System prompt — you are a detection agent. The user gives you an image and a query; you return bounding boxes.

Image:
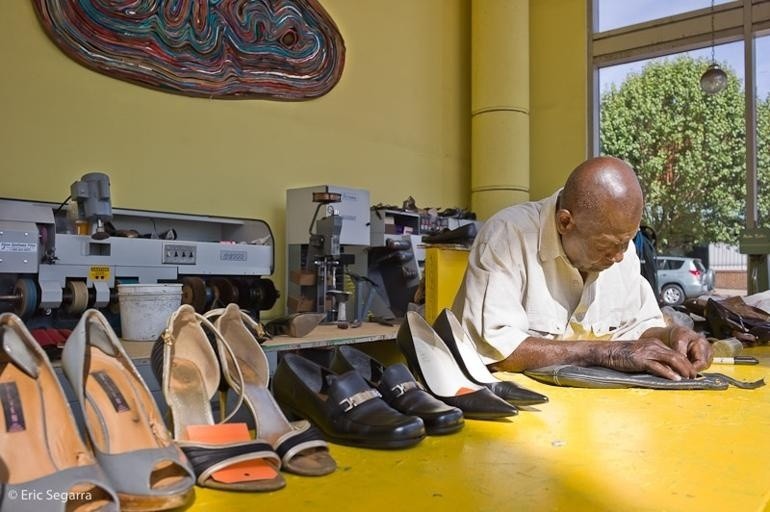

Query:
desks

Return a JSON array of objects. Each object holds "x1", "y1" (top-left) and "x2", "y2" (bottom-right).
[{"x1": 4, "y1": 320, "x2": 404, "y2": 458}]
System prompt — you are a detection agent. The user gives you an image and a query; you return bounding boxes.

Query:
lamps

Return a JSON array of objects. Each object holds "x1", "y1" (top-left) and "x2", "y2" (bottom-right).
[{"x1": 700, "y1": 1, "x2": 727, "y2": 94}]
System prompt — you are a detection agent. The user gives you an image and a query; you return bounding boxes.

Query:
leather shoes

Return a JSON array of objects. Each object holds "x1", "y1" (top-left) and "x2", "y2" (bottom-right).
[
  {"x1": 403, "y1": 195, "x2": 477, "y2": 219},
  {"x1": 272, "y1": 352, "x2": 427, "y2": 450},
  {"x1": 329, "y1": 345, "x2": 465, "y2": 435},
  {"x1": 420, "y1": 223, "x2": 476, "y2": 249}
]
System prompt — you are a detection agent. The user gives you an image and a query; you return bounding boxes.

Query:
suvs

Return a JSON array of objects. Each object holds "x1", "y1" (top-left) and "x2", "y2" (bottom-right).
[{"x1": 654, "y1": 256, "x2": 715, "y2": 306}]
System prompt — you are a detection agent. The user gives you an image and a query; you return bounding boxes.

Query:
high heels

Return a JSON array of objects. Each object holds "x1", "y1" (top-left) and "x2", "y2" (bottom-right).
[
  {"x1": 151, "y1": 304, "x2": 287, "y2": 492},
  {"x1": 0, "y1": 310, "x2": 121, "y2": 512},
  {"x1": 61, "y1": 309, "x2": 196, "y2": 511},
  {"x1": 705, "y1": 298, "x2": 770, "y2": 342},
  {"x1": 396, "y1": 310, "x2": 519, "y2": 418},
  {"x1": 211, "y1": 303, "x2": 337, "y2": 476},
  {"x1": 264, "y1": 312, "x2": 328, "y2": 336},
  {"x1": 433, "y1": 309, "x2": 549, "y2": 405}
]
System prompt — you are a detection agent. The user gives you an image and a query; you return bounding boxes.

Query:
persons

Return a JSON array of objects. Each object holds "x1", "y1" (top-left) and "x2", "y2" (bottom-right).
[{"x1": 451, "y1": 156, "x2": 715, "y2": 381}]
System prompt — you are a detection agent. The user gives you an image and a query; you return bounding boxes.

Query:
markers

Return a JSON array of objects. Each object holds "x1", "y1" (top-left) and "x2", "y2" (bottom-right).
[{"x1": 710, "y1": 355, "x2": 760, "y2": 366}]
